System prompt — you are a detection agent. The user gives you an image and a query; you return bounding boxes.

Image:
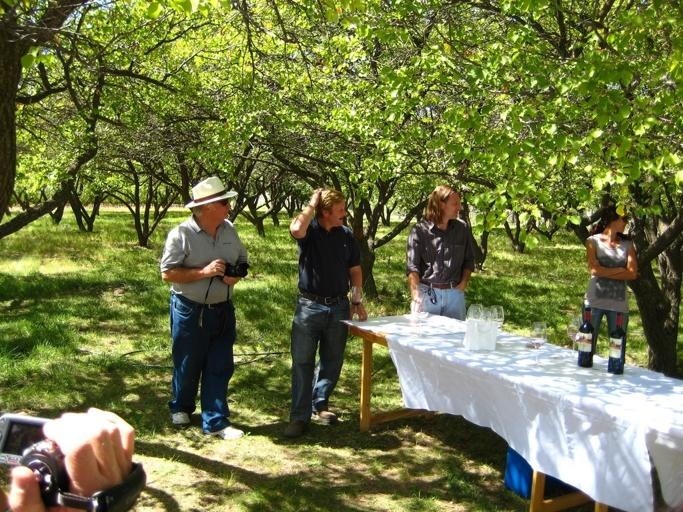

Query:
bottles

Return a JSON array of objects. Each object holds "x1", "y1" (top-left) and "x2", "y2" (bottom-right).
[
  {"x1": 607, "y1": 311, "x2": 626, "y2": 375},
  {"x1": 577, "y1": 307, "x2": 595, "y2": 368}
]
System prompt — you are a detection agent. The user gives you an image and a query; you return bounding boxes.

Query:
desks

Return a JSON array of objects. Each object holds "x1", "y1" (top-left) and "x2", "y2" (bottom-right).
[{"x1": 336, "y1": 309, "x2": 682, "y2": 511}]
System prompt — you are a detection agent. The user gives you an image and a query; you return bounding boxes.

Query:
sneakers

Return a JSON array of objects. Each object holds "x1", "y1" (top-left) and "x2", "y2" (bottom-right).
[
  {"x1": 172, "y1": 412, "x2": 191, "y2": 424},
  {"x1": 207, "y1": 426, "x2": 244, "y2": 439},
  {"x1": 283, "y1": 422, "x2": 305, "y2": 437},
  {"x1": 314, "y1": 411, "x2": 337, "y2": 420}
]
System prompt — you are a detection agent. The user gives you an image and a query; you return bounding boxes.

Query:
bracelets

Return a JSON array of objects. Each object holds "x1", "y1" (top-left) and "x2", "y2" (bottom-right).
[{"x1": 351, "y1": 301, "x2": 360, "y2": 306}]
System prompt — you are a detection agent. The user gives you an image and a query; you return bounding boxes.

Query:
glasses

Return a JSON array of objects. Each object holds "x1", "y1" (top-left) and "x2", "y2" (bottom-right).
[{"x1": 220, "y1": 199, "x2": 229, "y2": 206}]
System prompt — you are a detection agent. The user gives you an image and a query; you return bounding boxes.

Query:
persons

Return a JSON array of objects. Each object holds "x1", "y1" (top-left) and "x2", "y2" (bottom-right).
[
  {"x1": 281, "y1": 187, "x2": 368, "y2": 438},
  {"x1": 406, "y1": 184, "x2": 475, "y2": 320},
  {"x1": 159, "y1": 175, "x2": 247, "y2": 440},
  {"x1": 584, "y1": 204, "x2": 637, "y2": 360},
  {"x1": 0, "y1": 406, "x2": 136, "y2": 511}
]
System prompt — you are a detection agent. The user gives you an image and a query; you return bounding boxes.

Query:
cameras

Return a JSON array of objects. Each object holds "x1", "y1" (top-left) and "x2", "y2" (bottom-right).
[
  {"x1": 0, "y1": 412, "x2": 69, "y2": 511},
  {"x1": 217, "y1": 262, "x2": 248, "y2": 280}
]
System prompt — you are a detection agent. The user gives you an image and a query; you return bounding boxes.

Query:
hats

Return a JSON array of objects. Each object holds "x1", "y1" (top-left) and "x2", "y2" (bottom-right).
[{"x1": 185, "y1": 176, "x2": 238, "y2": 208}]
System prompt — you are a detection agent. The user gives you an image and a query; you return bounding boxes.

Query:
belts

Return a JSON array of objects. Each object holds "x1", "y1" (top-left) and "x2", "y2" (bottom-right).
[
  {"x1": 421, "y1": 281, "x2": 459, "y2": 288},
  {"x1": 178, "y1": 295, "x2": 228, "y2": 309},
  {"x1": 303, "y1": 291, "x2": 346, "y2": 305}
]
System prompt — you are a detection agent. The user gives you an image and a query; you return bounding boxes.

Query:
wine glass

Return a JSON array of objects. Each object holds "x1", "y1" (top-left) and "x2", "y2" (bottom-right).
[
  {"x1": 462, "y1": 304, "x2": 504, "y2": 355},
  {"x1": 528, "y1": 321, "x2": 547, "y2": 368},
  {"x1": 567, "y1": 320, "x2": 581, "y2": 363}
]
